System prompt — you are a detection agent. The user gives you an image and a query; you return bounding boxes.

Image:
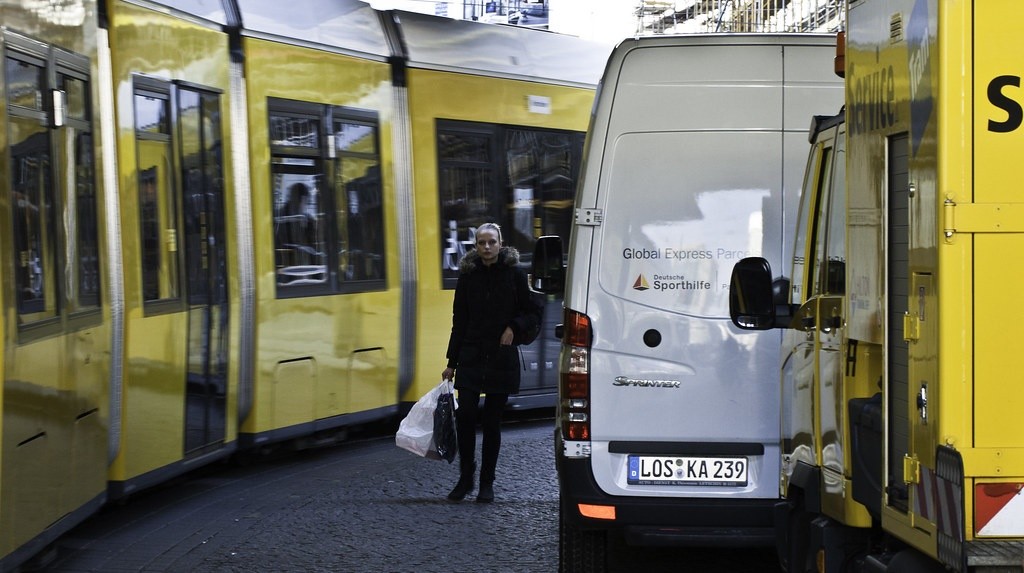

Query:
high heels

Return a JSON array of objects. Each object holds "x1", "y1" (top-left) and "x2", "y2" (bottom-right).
[
  {"x1": 478, "y1": 468, "x2": 496, "y2": 502},
  {"x1": 448, "y1": 464, "x2": 477, "y2": 500}
]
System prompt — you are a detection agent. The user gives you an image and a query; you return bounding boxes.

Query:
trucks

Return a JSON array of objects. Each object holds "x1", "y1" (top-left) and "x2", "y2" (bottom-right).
[{"x1": 729, "y1": 0, "x2": 1023, "y2": 573}]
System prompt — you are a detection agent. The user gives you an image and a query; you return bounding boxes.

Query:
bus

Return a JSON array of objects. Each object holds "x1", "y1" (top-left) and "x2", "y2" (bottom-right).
[{"x1": 0, "y1": 3, "x2": 613, "y2": 572}]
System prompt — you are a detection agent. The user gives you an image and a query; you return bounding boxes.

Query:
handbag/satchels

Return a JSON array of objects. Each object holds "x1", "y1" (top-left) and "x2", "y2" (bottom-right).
[{"x1": 395, "y1": 379, "x2": 458, "y2": 463}]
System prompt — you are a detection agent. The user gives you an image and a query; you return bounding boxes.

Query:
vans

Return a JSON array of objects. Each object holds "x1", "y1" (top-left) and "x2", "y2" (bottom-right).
[{"x1": 531, "y1": 33, "x2": 847, "y2": 573}]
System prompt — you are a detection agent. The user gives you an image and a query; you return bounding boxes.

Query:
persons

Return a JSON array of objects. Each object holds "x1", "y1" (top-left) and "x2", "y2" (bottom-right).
[{"x1": 440, "y1": 223, "x2": 544, "y2": 507}]
